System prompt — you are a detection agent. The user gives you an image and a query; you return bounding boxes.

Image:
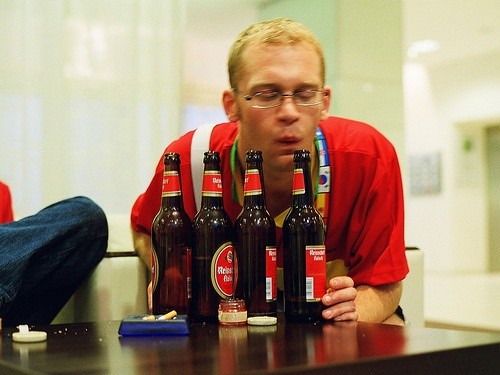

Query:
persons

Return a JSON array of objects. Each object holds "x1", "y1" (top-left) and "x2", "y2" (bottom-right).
[
  {"x1": 131, "y1": 18, "x2": 411, "y2": 321},
  {"x1": 0, "y1": 181, "x2": 109, "y2": 329}
]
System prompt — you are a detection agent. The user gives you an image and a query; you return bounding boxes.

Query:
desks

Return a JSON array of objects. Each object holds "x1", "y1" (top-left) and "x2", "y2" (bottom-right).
[{"x1": 0, "y1": 316, "x2": 500, "y2": 375}]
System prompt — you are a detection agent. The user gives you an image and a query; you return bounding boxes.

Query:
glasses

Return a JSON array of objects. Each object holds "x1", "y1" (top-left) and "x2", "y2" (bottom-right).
[{"x1": 236, "y1": 88, "x2": 327, "y2": 109}]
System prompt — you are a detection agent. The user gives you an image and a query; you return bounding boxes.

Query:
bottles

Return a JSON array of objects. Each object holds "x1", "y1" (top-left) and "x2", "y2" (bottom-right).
[
  {"x1": 281, "y1": 150, "x2": 327, "y2": 326},
  {"x1": 233, "y1": 149, "x2": 279, "y2": 319},
  {"x1": 192, "y1": 151, "x2": 238, "y2": 325},
  {"x1": 150, "y1": 153, "x2": 195, "y2": 323}
]
